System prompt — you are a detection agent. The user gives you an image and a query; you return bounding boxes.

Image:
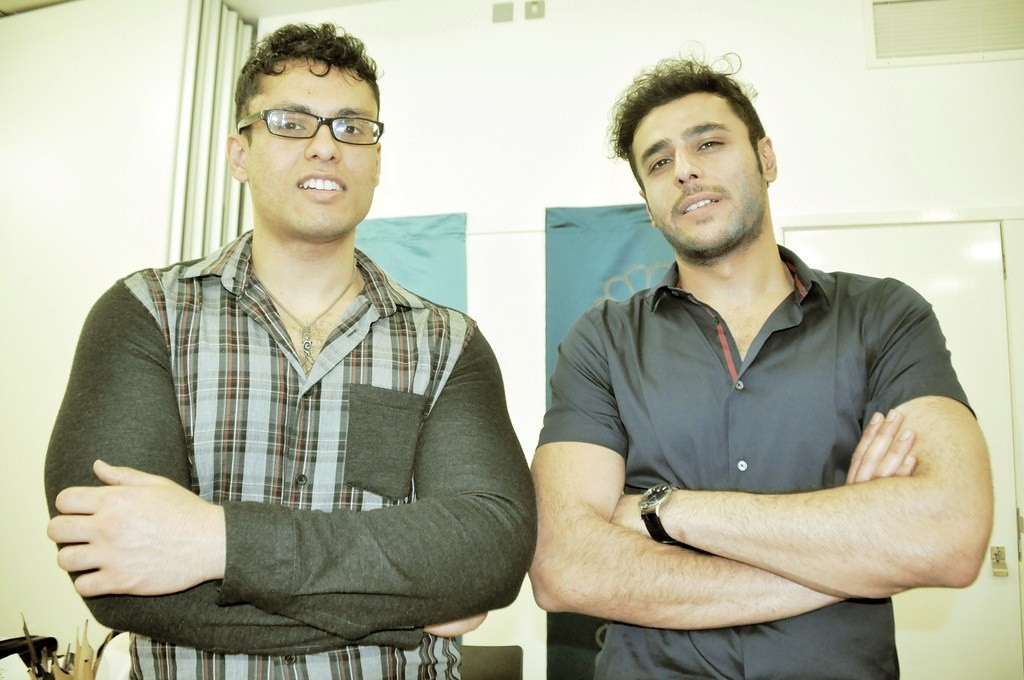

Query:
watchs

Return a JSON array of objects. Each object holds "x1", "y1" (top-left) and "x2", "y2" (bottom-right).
[{"x1": 638, "y1": 485, "x2": 679, "y2": 545}]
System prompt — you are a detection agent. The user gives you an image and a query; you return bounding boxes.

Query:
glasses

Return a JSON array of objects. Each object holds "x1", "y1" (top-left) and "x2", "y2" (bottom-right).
[{"x1": 238, "y1": 109, "x2": 384, "y2": 145}]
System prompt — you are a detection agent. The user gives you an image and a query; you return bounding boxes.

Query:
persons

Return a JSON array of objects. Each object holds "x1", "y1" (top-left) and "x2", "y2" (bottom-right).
[
  {"x1": 528, "y1": 62, "x2": 996, "y2": 680},
  {"x1": 44, "y1": 22, "x2": 537, "y2": 680}
]
